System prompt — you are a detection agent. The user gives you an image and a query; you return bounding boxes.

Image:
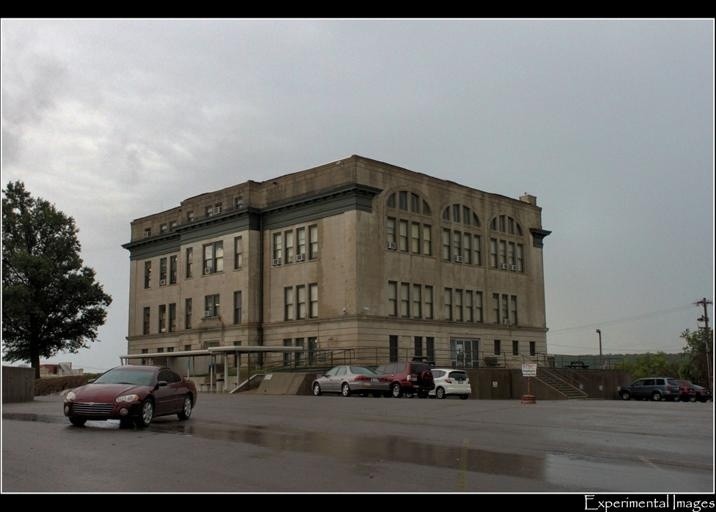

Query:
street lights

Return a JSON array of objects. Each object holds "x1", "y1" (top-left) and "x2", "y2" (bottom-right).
[{"x1": 596, "y1": 328, "x2": 602, "y2": 368}]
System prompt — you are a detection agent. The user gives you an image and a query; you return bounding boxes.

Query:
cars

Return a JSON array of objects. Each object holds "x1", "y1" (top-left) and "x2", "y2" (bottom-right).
[
  {"x1": 618, "y1": 377, "x2": 711, "y2": 402},
  {"x1": 564, "y1": 360, "x2": 589, "y2": 368},
  {"x1": 311, "y1": 362, "x2": 472, "y2": 399},
  {"x1": 64, "y1": 364, "x2": 198, "y2": 428}
]
